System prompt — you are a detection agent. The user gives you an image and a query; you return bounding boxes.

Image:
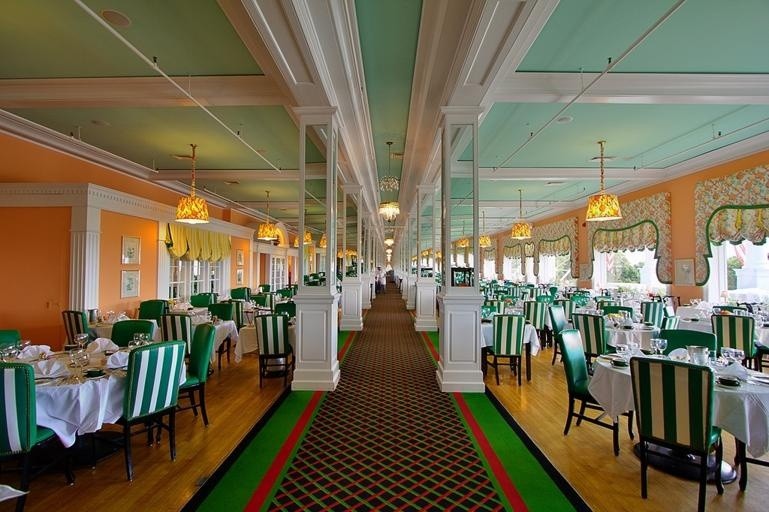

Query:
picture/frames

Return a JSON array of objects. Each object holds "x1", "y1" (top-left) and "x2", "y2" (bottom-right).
[
  {"x1": 121, "y1": 236, "x2": 141, "y2": 265},
  {"x1": 236, "y1": 249, "x2": 244, "y2": 266}
]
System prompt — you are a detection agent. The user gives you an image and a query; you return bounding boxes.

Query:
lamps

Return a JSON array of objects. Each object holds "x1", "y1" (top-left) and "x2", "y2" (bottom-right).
[
  {"x1": 455, "y1": 211, "x2": 491, "y2": 250},
  {"x1": 257, "y1": 190, "x2": 278, "y2": 241},
  {"x1": 294, "y1": 210, "x2": 326, "y2": 248},
  {"x1": 510, "y1": 189, "x2": 533, "y2": 240},
  {"x1": 176, "y1": 145, "x2": 211, "y2": 223},
  {"x1": 378, "y1": 142, "x2": 400, "y2": 224},
  {"x1": 585, "y1": 141, "x2": 623, "y2": 222}
]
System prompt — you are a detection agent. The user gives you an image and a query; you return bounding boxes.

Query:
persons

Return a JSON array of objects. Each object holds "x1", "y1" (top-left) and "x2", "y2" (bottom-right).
[{"x1": 376, "y1": 264, "x2": 388, "y2": 294}]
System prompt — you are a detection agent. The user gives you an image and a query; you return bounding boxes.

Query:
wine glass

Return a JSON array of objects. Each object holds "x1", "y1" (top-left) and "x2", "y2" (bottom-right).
[
  {"x1": 609, "y1": 338, "x2": 745, "y2": 390},
  {"x1": 0, "y1": 333, "x2": 151, "y2": 380}
]
